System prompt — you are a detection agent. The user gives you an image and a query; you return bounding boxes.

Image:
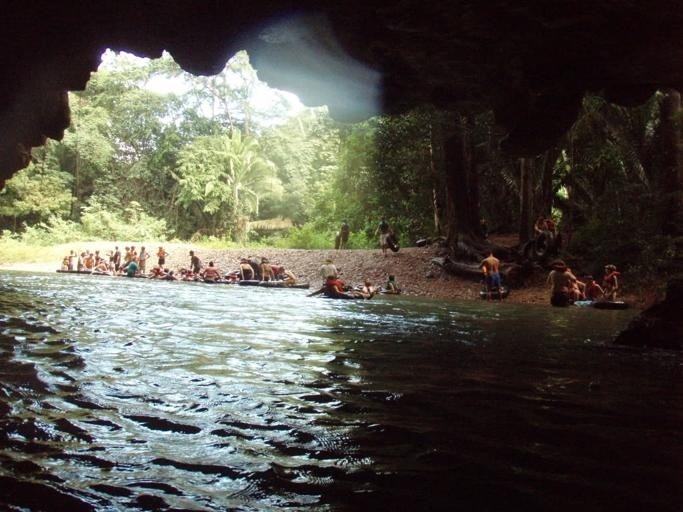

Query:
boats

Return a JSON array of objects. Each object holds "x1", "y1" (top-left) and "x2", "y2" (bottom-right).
[{"x1": 54, "y1": 267, "x2": 311, "y2": 288}]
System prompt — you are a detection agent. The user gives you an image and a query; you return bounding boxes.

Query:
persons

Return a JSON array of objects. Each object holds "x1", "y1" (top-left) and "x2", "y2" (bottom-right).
[
  {"x1": 374, "y1": 215, "x2": 393, "y2": 257},
  {"x1": 336, "y1": 276, "x2": 346, "y2": 292},
  {"x1": 478, "y1": 248, "x2": 503, "y2": 303},
  {"x1": 544, "y1": 258, "x2": 577, "y2": 306},
  {"x1": 566, "y1": 264, "x2": 620, "y2": 305},
  {"x1": 325, "y1": 275, "x2": 347, "y2": 299},
  {"x1": 501, "y1": 215, "x2": 557, "y2": 288},
  {"x1": 380, "y1": 274, "x2": 400, "y2": 294},
  {"x1": 339, "y1": 220, "x2": 349, "y2": 249},
  {"x1": 61, "y1": 246, "x2": 295, "y2": 287},
  {"x1": 360, "y1": 278, "x2": 374, "y2": 300},
  {"x1": 318, "y1": 257, "x2": 338, "y2": 284},
  {"x1": 334, "y1": 232, "x2": 341, "y2": 250}
]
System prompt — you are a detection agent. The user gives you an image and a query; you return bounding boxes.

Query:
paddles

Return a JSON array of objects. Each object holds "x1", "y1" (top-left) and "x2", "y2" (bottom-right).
[{"x1": 306, "y1": 287, "x2": 326, "y2": 296}]
